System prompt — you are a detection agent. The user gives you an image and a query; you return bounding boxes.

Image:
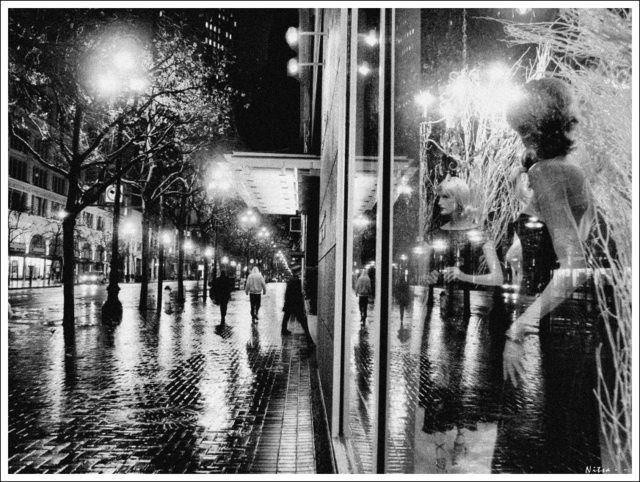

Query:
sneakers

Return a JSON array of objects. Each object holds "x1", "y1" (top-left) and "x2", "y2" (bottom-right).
[
  {"x1": 281, "y1": 329, "x2": 292, "y2": 335},
  {"x1": 252, "y1": 315, "x2": 259, "y2": 323}
]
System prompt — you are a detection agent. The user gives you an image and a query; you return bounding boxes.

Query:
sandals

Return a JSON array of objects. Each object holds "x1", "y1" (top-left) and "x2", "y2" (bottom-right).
[
  {"x1": 453, "y1": 430, "x2": 470, "y2": 466},
  {"x1": 434, "y1": 442, "x2": 448, "y2": 474}
]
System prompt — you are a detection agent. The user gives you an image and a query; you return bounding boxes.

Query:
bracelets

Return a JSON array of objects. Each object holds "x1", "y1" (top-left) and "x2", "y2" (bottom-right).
[{"x1": 503, "y1": 329, "x2": 526, "y2": 346}]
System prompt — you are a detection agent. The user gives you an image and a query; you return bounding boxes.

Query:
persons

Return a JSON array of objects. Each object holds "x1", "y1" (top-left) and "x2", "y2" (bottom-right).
[
  {"x1": 352, "y1": 265, "x2": 373, "y2": 329},
  {"x1": 415, "y1": 171, "x2": 505, "y2": 474},
  {"x1": 393, "y1": 274, "x2": 412, "y2": 326},
  {"x1": 211, "y1": 271, "x2": 235, "y2": 327},
  {"x1": 278, "y1": 264, "x2": 311, "y2": 337},
  {"x1": 244, "y1": 264, "x2": 267, "y2": 323},
  {"x1": 487, "y1": 74, "x2": 609, "y2": 475}
]
